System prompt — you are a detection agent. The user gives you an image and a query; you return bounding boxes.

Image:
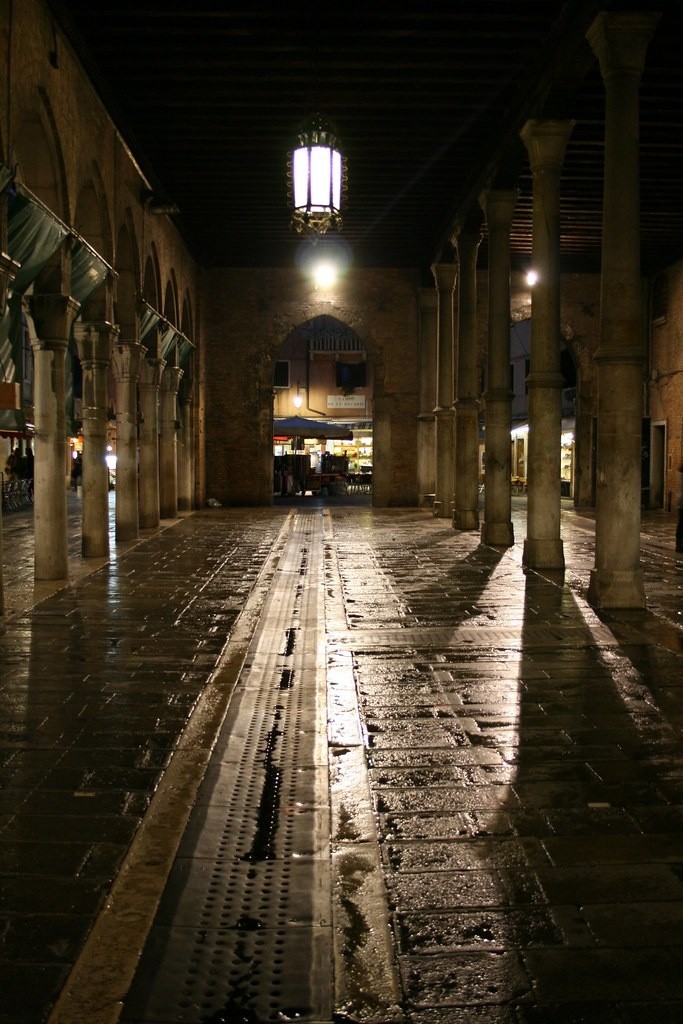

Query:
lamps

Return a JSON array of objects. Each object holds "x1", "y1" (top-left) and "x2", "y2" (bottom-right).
[{"x1": 286, "y1": 110, "x2": 349, "y2": 235}]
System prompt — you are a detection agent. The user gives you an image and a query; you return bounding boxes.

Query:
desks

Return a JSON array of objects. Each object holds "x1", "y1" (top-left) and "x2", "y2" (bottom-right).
[{"x1": 312, "y1": 473, "x2": 339, "y2": 496}]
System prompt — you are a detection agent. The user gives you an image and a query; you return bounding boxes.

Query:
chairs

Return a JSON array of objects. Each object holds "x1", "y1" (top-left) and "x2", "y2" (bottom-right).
[{"x1": 346, "y1": 474, "x2": 373, "y2": 495}]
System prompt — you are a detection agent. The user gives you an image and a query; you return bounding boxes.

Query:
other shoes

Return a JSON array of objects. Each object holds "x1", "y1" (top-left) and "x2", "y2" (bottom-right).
[{"x1": 72, "y1": 487, "x2": 76, "y2": 491}]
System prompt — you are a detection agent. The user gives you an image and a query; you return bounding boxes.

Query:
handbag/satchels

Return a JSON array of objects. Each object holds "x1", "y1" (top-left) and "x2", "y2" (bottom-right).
[{"x1": 8, "y1": 467, "x2": 13, "y2": 475}]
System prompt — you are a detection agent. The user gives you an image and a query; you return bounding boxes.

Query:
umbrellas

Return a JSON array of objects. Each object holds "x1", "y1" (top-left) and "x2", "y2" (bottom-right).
[{"x1": 274, "y1": 416, "x2": 353, "y2": 469}]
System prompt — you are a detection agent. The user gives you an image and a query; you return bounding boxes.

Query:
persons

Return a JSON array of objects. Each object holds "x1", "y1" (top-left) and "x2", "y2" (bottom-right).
[
  {"x1": 7, "y1": 448, "x2": 34, "y2": 496},
  {"x1": 72, "y1": 454, "x2": 81, "y2": 491},
  {"x1": 512, "y1": 476, "x2": 522, "y2": 495},
  {"x1": 342, "y1": 450, "x2": 349, "y2": 470}
]
